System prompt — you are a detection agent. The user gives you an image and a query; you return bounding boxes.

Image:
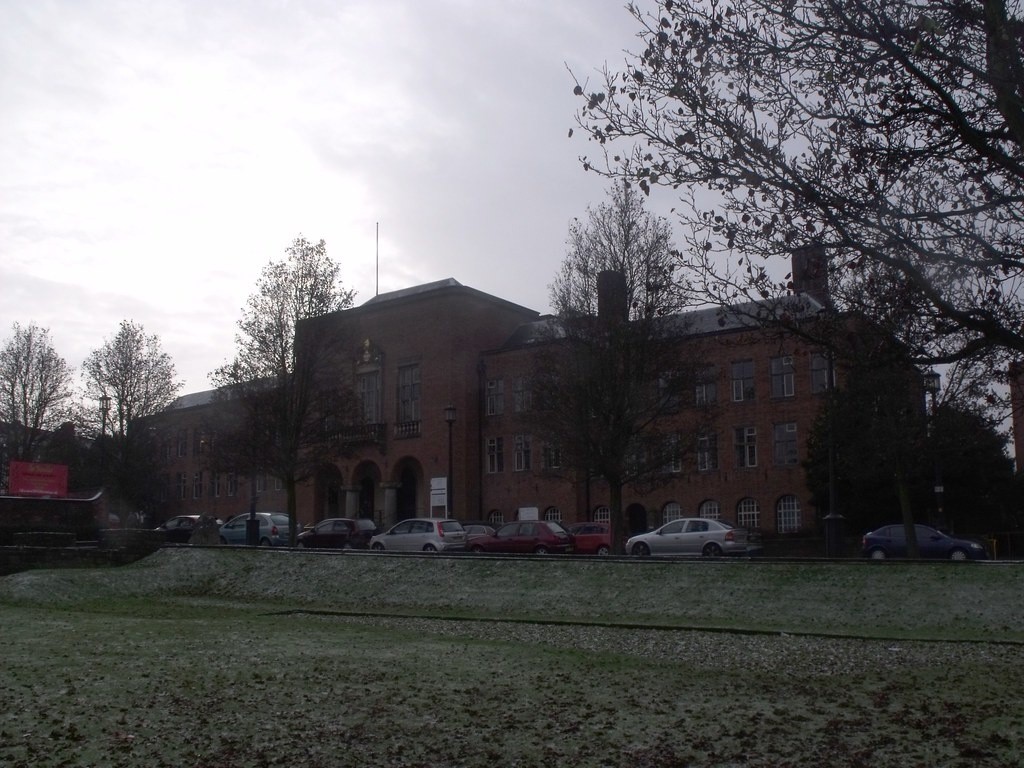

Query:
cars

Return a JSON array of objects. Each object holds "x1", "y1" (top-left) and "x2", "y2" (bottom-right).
[
  {"x1": 369, "y1": 516, "x2": 468, "y2": 552},
  {"x1": 465, "y1": 519, "x2": 577, "y2": 556},
  {"x1": 567, "y1": 522, "x2": 630, "y2": 557},
  {"x1": 219, "y1": 513, "x2": 301, "y2": 547},
  {"x1": 625, "y1": 517, "x2": 749, "y2": 560},
  {"x1": 462, "y1": 526, "x2": 498, "y2": 540},
  {"x1": 296, "y1": 517, "x2": 381, "y2": 549},
  {"x1": 862, "y1": 525, "x2": 987, "y2": 562},
  {"x1": 154, "y1": 514, "x2": 224, "y2": 542}
]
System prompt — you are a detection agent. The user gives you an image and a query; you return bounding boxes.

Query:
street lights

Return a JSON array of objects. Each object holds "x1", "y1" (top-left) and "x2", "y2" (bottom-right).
[
  {"x1": 925, "y1": 365, "x2": 942, "y2": 422},
  {"x1": 98, "y1": 396, "x2": 113, "y2": 485},
  {"x1": 818, "y1": 303, "x2": 849, "y2": 559},
  {"x1": 443, "y1": 404, "x2": 458, "y2": 518},
  {"x1": 245, "y1": 401, "x2": 264, "y2": 546}
]
